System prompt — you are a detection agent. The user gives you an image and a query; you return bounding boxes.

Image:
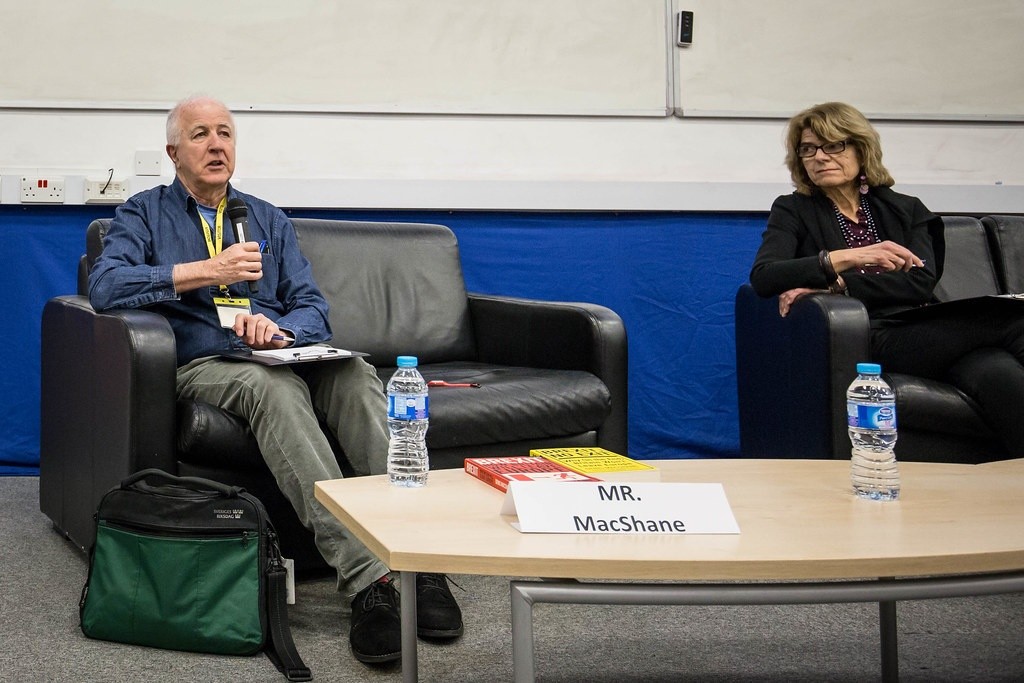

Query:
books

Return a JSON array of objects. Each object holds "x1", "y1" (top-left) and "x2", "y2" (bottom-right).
[
  {"x1": 464, "y1": 456, "x2": 606, "y2": 494},
  {"x1": 530, "y1": 447, "x2": 661, "y2": 482}
]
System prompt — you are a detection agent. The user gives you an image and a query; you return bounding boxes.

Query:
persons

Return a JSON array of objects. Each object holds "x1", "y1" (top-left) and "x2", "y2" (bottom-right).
[
  {"x1": 747, "y1": 102, "x2": 1024, "y2": 461},
  {"x1": 87, "y1": 96, "x2": 464, "y2": 662}
]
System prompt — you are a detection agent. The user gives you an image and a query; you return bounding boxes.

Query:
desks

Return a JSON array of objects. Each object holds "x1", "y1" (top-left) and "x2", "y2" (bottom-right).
[{"x1": 315, "y1": 457, "x2": 1023, "y2": 683}]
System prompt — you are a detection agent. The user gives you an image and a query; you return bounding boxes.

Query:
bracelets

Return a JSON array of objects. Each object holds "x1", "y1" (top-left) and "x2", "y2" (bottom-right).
[
  {"x1": 819, "y1": 249, "x2": 838, "y2": 281},
  {"x1": 831, "y1": 279, "x2": 841, "y2": 293}
]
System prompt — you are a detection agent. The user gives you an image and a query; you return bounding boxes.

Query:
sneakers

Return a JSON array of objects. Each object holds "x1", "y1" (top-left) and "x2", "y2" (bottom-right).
[
  {"x1": 347, "y1": 578, "x2": 402, "y2": 664},
  {"x1": 415, "y1": 571, "x2": 468, "y2": 639}
]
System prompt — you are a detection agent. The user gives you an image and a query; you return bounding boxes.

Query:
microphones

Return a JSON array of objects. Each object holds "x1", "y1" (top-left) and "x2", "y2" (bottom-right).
[{"x1": 227, "y1": 198, "x2": 260, "y2": 293}]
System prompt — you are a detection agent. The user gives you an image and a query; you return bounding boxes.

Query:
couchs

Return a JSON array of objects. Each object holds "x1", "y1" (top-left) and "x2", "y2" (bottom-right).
[
  {"x1": 735, "y1": 215, "x2": 1024, "y2": 458},
  {"x1": 40, "y1": 218, "x2": 628, "y2": 582}
]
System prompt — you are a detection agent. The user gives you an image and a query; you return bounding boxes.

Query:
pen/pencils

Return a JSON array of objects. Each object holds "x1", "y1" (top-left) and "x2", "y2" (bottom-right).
[
  {"x1": 864, "y1": 259, "x2": 926, "y2": 267},
  {"x1": 271, "y1": 335, "x2": 295, "y2": 341},
  {"x1": 260, "y1": 240, "x2": 267, "y2": 253}
]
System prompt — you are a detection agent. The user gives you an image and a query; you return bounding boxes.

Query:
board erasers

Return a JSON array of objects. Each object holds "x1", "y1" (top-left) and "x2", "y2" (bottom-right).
[{"x1": 677, "y1": 11, "x2": 694, "y2": 47}]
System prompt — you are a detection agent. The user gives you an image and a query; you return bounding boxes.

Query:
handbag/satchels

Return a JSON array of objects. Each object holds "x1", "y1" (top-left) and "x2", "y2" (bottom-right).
[{"x1": 78, "y1": 468, "x2": 313, "y2": 682}]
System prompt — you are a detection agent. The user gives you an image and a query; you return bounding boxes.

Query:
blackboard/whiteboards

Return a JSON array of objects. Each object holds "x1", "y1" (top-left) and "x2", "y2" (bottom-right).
[
  {"x1": 672, "y1": 0, "x2": 1024, "y2": 122},
  {"x1": 0, "y1": 0, "x2": 673, "y2": 117}
]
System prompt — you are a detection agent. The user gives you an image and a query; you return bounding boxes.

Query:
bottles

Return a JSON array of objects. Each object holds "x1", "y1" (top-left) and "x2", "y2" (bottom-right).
[
  {"x1": 387, "y1": 356, "x2": 429, "y2": 487},
  {"x1": 847, "y1": 363, "x2": 901, "y2": 501}
]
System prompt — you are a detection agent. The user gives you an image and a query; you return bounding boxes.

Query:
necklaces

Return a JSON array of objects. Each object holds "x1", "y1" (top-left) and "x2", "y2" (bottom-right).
[{"x1": 833, "y1": 195, "x2": 881, "y2": 249}]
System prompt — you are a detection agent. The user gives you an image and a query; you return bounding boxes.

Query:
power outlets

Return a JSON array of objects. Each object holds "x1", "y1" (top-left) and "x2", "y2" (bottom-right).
[{"x1": 21, "y1": 177, "x2": 65, "y2": 203}]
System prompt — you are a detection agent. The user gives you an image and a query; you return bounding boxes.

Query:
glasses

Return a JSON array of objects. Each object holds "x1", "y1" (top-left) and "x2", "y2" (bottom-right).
[{"x1": 795, "y1": 140, "x2": 852, "y2": 158}]
